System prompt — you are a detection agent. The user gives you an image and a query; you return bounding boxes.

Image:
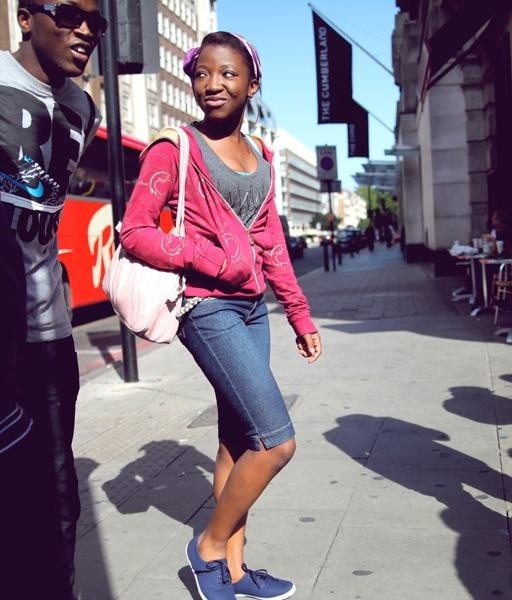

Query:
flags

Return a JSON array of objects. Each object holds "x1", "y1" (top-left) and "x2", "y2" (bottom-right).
[
  {"x1": 349, "y1": 99, "x2": 369, "y2": 158},
  {"x1": 312, "y1": 10, "x2": 354, "y2": 123}
]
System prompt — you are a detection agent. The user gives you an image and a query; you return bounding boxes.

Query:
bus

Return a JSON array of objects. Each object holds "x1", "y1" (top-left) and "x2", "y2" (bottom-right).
[{"x1": 56, "y1": 126, "x2": 174, "y2": 322}]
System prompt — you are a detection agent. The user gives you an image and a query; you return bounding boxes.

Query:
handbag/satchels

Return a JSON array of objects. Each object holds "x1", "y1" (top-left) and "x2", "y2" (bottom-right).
[{"x1": 101, "y1": 126, "x2": 189, "y2": 345}]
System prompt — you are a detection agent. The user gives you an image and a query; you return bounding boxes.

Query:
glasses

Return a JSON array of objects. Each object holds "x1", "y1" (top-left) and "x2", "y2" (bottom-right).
[{"x1": 52, "y1": 2, "x2": 110, "y2": 40}]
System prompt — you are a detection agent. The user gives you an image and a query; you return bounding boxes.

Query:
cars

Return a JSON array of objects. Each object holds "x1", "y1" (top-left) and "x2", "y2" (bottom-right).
[{"x1": 278, "y1": 215, "x2": 367, "y2": 262}]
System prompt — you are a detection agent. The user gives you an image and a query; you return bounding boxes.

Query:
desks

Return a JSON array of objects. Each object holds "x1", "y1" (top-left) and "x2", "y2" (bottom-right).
[{"x1": 449, "y1": 249, "x2": 512, "y2": 343}]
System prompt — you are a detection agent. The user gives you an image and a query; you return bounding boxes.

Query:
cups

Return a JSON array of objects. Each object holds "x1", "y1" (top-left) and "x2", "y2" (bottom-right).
[{"x1": 496, "y1": 240, "x2": 504, "y2": 254}]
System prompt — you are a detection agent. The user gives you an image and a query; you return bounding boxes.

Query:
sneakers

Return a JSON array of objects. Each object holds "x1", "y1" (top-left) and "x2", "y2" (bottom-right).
[
  {"x1": 233, "y1": 563, "x2": 296, "y2": 600},
  {"x1": 185, "y1": 536, "x2": 237, "y2": 600}
]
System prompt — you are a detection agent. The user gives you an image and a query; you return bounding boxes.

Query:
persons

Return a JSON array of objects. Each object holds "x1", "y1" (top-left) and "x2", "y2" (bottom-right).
[
  {"x1": 452, "y1": 209, "x2": 508, "y2": 297},
  {"x1": 118, "y1": 31, "x2": 322, "y2": 600},
  {"x1": 385, "y1": 225, "x2": 393, "y2": 247},
  {"x1": 365, "y1": 225, "x2": 374, "y2": 252},
  {"x1": 0, "y1": 2, "x2": 108, "y2": 600}
]
